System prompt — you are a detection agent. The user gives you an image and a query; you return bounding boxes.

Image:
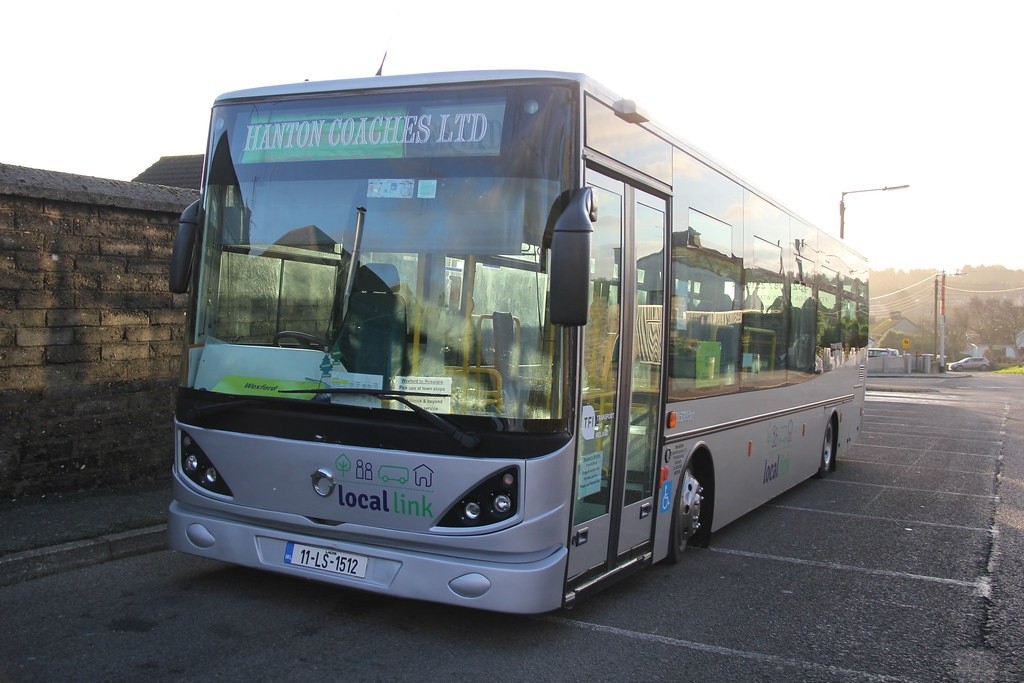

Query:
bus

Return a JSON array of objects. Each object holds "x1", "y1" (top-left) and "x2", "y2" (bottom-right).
[
  {"x1": 868, "y1": 348, "x2": 899, "y2": 357},
  {"x1": 169, "y1": 68, "x2": 869, "y2": 618}
]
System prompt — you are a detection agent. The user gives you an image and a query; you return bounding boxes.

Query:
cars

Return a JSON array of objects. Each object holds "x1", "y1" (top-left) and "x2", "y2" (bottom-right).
[{"x1": 950, "y1": 357, "x2": 990, "y2": 372}]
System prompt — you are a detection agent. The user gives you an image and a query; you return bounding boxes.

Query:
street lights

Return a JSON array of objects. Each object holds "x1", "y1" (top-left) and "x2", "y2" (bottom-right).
[
  {"x1": 839, "y1": 185, "x2": 909, "y2": 238},
  {"x1": 932, "y1": 272, "x2": 968, "y2": 362}
]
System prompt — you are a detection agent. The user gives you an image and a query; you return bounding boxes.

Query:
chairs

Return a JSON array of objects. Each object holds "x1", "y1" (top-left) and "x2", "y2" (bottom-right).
[{"x1": 333, "y1": 263, "x2": 403, "y2": 377}]
